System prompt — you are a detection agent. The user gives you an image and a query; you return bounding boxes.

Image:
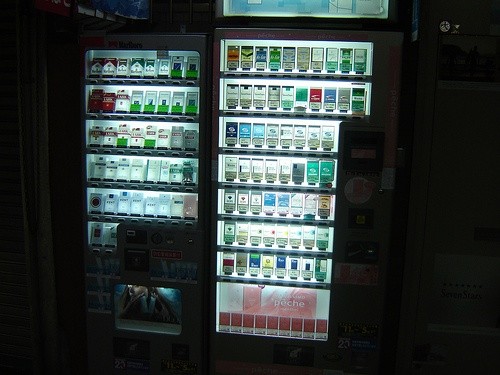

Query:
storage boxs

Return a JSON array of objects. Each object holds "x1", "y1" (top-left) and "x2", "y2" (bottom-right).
[
  {"x1": 88, "y1": 56, "x2": 200, "y2": 245},
  {"x1": 223, "y1": 46, "x2": 366, "y2": 282}
]
